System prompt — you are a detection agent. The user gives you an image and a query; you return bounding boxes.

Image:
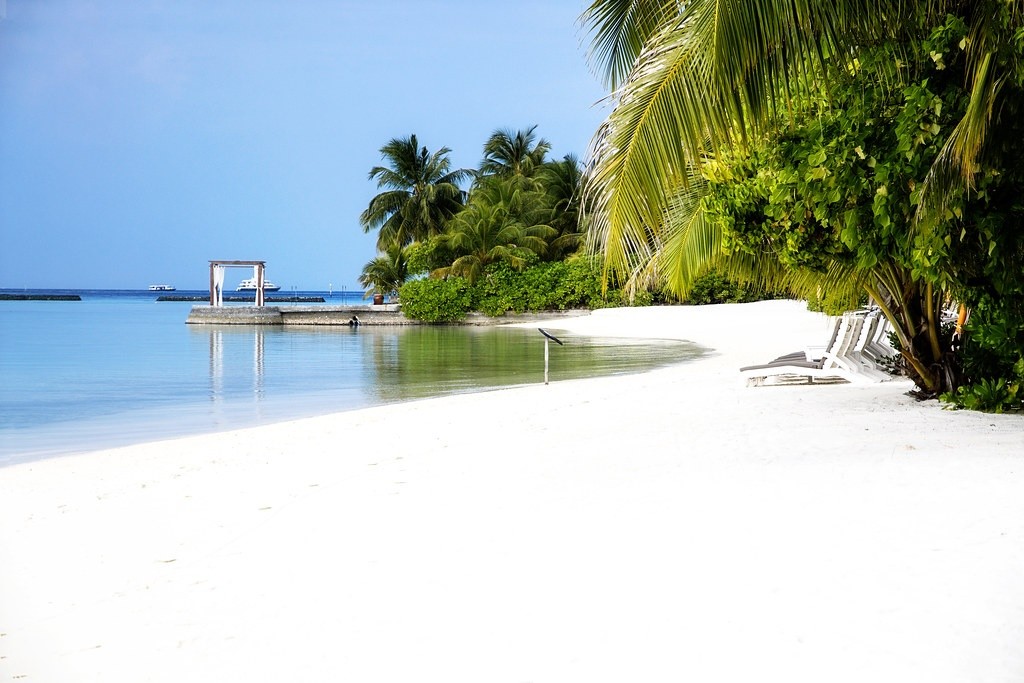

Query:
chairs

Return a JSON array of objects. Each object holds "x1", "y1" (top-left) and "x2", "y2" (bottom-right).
[{"x1": 740, "y1": 309, "x2": 896, "y2": 389}]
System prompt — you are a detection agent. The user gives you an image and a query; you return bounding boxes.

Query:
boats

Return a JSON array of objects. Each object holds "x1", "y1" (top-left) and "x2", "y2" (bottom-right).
[
  {"x1": 149, "y1": 285, "x2": 176, "y2": 291},
  {"x1": 236, "y1": 276, "x2": 281, "y2": 292}
]
